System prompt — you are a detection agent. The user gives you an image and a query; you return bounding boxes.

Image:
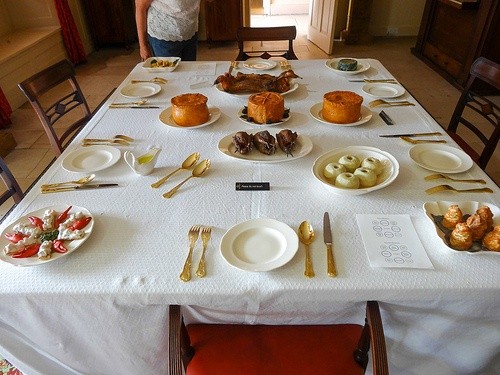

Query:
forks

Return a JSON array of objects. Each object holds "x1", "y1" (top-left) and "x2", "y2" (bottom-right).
[
  {"x1": 419, "y1": 173, "x2": 494, "y2": 195},
  {"x1": 179, "y1": 226, "x2": 212, "y2": 284},
  {"x1": 81, "y1": 133, "x2": 134, "y2": 146}
]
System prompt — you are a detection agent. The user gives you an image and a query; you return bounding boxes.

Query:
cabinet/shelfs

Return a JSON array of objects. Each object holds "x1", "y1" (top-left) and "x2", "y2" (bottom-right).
[{"x1": 410, "y1": 0, "x2": 500, "y2": 96}]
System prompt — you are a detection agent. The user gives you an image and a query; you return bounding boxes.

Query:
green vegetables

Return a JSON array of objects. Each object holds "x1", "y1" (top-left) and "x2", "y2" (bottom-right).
[{"x1": 40, "y1": 228, "x2": 59, "y2": 241}]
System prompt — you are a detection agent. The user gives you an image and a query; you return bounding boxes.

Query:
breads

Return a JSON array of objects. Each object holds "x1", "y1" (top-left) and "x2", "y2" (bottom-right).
[
  {"x1": 323, "y1": 155, "x2": 383, "y2": 189},
  {"x1": 247, "y1": 91, "x2": 284, "y2": 123},
  {"x1": 171, "y1": 93, "x2": 210, "y2": 127},
  {"x1": 337, "y1": 59, "x2": 357, "y2": 72},
  {"x1": 442, "y1": 204, "x2": 500, "y2": 250},
  {"x1": 322, "y1": 91, "x2": 363, "y2": 124}
]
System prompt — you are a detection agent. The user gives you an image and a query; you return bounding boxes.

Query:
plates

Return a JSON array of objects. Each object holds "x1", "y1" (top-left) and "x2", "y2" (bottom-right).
[
  {"x1": 219, "y1": 218, "x2": 300, "y2": 272},
  {"x1": 212, "y1": 57, "x2": 313, "y2": 163},
  {"x1": 158, "y1": 103, "x2": 222, "y2": 129},
  {"x1": 309, "y1": 102, "x2": 373, "y2": 126},
  {"x1": 407, "y1": 142, "x2": 474, "y2": 174},
  {"x1": 362, "y1": 82, "x2": 405, "y2": 98},
  {"x1": 0, "y1": 204, "x2": 94, "y2": 266},
  {"x1": 325, "y1": 57, "x2": 370, "y2": 74},
  {"x1": 423, "y1": 199, "x2": 500, "y2": 255},
  {"x1": 311, "y1": 145, "x2": 400, "y2": 196},
  {"x1": 140, "y1": 56, "x2": 181, "y2": 72},
  {"x1": 61, "y1": 144, "x2": 121, "y2": 173},
  {"x1": 119, "y1": 82, "x2": 162, "y2": 97}
]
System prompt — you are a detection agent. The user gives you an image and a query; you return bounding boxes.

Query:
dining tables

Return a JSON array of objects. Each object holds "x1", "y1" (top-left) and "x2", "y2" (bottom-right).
[{"x1": 0, "y1": 59, "x2": 500, "y2": 375}]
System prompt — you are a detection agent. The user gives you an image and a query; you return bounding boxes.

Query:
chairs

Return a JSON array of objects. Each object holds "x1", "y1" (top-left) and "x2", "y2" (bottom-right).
[
  {"x1": 169, "y1": 301, "x2": 389, "y2": 375},
  {"x1": 0, "y1": 157, "x2": 25, "y2": 224},
  {"x1": 18, "y1": 58, "x2": 91, "y2": 159},
  {"x1": 446, "y1": 57, "x2": 500, "y2": 171},
  {"x1": 235, "y1": 26, "x2": 297, "y2": 61}
]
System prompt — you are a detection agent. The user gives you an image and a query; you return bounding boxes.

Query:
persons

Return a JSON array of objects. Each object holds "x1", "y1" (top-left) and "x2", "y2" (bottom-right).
[{"x1": 135, "y1": 0, "x2": 200, "y2": 62}]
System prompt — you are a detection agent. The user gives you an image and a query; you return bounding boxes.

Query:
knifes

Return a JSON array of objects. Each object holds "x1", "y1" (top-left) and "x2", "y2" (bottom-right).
[
  {"x1": 109, "y1": 105, "x2": 159, "y2": 109},
  {"x1": 380, "y1": 132, "x2": 441, "y2": 139},
  {"x1": 322, "y1": 212, "x2": 338, "y2": 279},
  {"x1": 41, "y1": 183, "x2": 119, "y2": 195}
]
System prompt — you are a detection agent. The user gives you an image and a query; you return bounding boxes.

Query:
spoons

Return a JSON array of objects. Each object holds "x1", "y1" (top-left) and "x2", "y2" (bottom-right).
[
  {"x1": 41, "y1": 174, "x2": 96, "y2": 188},
  {"x1": 296, "y1": 221, "x2": 317, "y2": 277},
  {"x1": 150, "y1": 152, "x2": 212, "y2": 198},
  {"x1": 400, "y1": 135, "x2": 447, "y2": 145}
]
